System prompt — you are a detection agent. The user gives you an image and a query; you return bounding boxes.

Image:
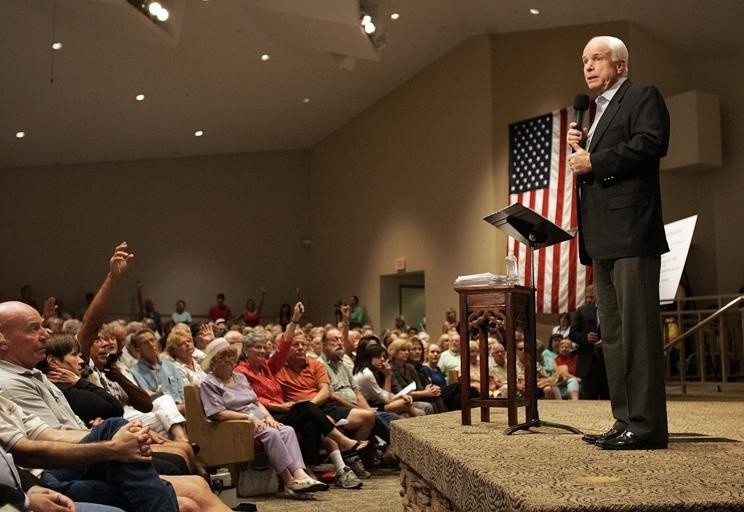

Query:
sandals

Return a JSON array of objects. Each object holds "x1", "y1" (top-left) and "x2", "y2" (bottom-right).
[{"x1": 286, "y1": 478, "x2": 329, "y2": 495}]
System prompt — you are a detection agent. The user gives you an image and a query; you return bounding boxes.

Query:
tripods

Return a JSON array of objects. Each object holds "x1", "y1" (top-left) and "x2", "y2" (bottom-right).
[{"x1": 482, "y1": 202, "x2": 585, "y2": 436}]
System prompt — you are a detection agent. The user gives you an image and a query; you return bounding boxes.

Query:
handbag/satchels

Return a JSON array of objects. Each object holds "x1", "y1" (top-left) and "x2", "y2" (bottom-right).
[{"x1": 235, "y1": 455, "x2": 282, "y2": 499}]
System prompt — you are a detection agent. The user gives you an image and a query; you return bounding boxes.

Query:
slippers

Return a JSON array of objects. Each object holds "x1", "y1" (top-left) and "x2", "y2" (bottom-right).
[{"x1": 340, "y1": 440, "x2": 370, "y2": 459}]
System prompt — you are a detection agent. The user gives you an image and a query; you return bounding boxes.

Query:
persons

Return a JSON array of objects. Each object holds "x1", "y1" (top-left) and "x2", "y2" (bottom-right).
[
  {"x1": 350, "y1": 344, "x2": 413, "y2": 421},
  {"x1": 349, "y1": 295, "x2": 366, "y2": 328},
  {"x1": 408, "y1": 337, "x2": 432, "y2": 394},
  {"x1": 552, "y1": 312, "x2": 571, "y2": 338},
  {"x1": 99, "y1": 318, "x2": 165, "y2": 357},
  {"x1": 569, "y1": 287, "x2": 610, "y2": 400},
  {"x1": 436, "y1": 320, "x2": 465, "y2": 346},
  {"x1": 126, "y1": 327, "x2": 187, "y2": 415},
  {"x1": 136, "y1": 280, "x2": 162, "y2": 330},
  {"x1": 272, "y1": 330, "x2": 375, "y2": 489},
  {"x1": 442, "y1": 308, "x2": 460, "y2": 332},
  {"x1": 357, "y1": 335, "x2": 434, "y2": 417},
  {"x1": 199, "y1": 338, "x2": 330, "y2": 501},
  {"x1": 101, "y1": 326, "x2": 210, "y2": 480},
  {"x1": 540, "y1": 334, "x2": 582, "y2": 400},
  {"x1": 0, "y1": 441, "x2": 123, "y2": 511},
  {"x1": 232, "y1": 301, "x2": 370, "y2": 492},
  {"x1": 165, "y1": 329, "x2": 207, "y2": 414},
  {"x1": 1, "y1": 395, "x2": 179, "y2": 511},
  {"x1": 469, "y1": 340, "x2": 502, "y2": 400},
  {"x1": 568, "y1": 37, "x2": 669, "y2": 451},
  {"x1": 171, "y1": 320, "x2": 253, "y2": 354},
  {"x1": 553, "y1": 340, "x2": 579, "y2": 377},
  {"x1": 42, "y1": 317, "x2": 85, "y2": 351},
  {"x1": 276, "y1": 303, "x2": 292, "y2": 329},
  {"x1": 0, "y1": 300, "x2": 256, "y2": 510},
  {"x1": 168, "y1": 299, "x2": 193, "y2": 329},
  {"x1": 385, "y1": 339, "x2": 446, "y2": 414},
  {"x1": 36, "y1": 335, "x2": 191, "y2": 479},
  {"x1": 349, "y1": 316, "x2": 439, "y2": 347},
  {"x1": 208, "y1": 293, "x2": 233, "y2": 323},
  {"x1": 252, "y1": 322, "x2": 350, "y2": 352},
  {"x1": 438, "y1": 334, "x2": 462, "y2": 384},
  {"x1": 18, "y1": 285, "x2": 39, "y2": 314},
  {"x1": 425, "y1": 343, "x2": 481, "y2": 411},
  {"x1": 73, "y1": 242, "x2": 154, "y2": 414},
  {"x1": 488, "y1": 343, "x2": 527, "y2": 402},
  {"x1": 233, "y1": 287, "x2": 266, "y2": 327},
  {"x1": 313, "y1": 327, "x2": 402, "y2": 445}
]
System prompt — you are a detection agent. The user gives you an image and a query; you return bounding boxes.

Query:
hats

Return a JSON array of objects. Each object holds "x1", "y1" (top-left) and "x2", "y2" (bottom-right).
[{"x1": 203, "y1": 338, "x2": 243, "y2": 374}]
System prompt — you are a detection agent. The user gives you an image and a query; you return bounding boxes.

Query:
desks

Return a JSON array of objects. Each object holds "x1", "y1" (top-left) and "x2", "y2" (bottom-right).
[{"x1": 451, "y1": 284, "x2": 540, "y2": 428}]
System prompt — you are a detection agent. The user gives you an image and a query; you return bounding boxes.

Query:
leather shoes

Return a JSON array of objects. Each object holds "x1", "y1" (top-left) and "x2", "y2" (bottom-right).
[
  {"x1": 579, "y1": 424, "x2": 630, "y2": 440},
  {"x1": 585, "y1": 428, "x2": 670, "y2": 448}
]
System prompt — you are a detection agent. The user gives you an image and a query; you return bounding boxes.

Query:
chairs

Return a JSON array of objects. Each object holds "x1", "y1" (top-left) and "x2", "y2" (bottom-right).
[{"x1": 183, "y1": 384, "x2": 257, "y2": 468}]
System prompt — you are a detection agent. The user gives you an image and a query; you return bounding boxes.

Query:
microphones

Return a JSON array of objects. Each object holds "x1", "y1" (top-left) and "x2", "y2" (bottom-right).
[{"x1": 569, "y1": 95, "x2": 591, "y2": 150}]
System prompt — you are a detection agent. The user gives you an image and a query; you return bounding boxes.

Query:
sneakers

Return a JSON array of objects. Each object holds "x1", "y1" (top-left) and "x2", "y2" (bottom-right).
[
  {"x1": 333, "y1": 469, "x2": 363, "y2": 488},
  {"x1": 348, "y1": 458, "x2": 372, "y2": 479}
]
